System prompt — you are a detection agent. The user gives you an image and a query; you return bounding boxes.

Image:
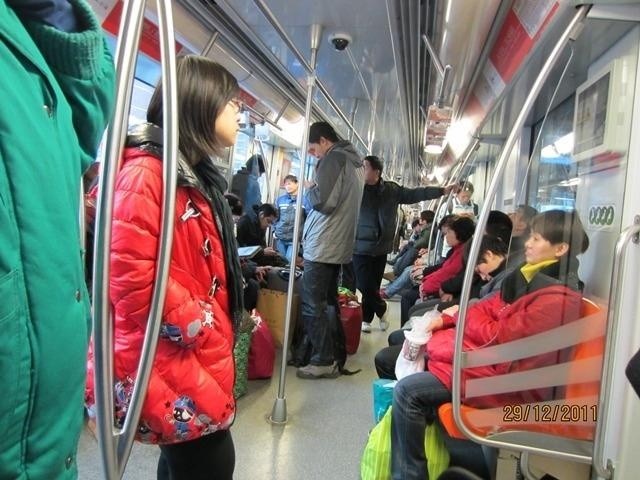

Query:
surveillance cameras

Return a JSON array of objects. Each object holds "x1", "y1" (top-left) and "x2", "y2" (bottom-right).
[{"x1": 328, "y1": 33, "x2": 352, "y2": 51}]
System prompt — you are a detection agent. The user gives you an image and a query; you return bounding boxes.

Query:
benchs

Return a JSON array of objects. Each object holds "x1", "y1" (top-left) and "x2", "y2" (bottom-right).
[{"x1": 437, "y1": 296, "x2": 608, "y2": 480}]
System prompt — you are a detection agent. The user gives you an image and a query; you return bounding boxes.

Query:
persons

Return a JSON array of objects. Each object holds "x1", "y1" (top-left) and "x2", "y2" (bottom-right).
[
  {"x1": 228, "y1": 154, "x2": 290, "y2": 283},
  {"x1": 297, "y1": 122, "x2": 363, "y2": 379},
  {"x1": 352, "y1": 156, "x2": 459, "y2": 333},
  {"x1": 85, "y1": 54, "x2": 242, "y2": 480},
  {"x1": 375, "y1": 181, "x2": 539, "y2": 346},
  {"x1": 374, "y1": 210, "x2": 589, "y2": 480},
  {"x1": 271, "y1": 175, "x2": 299, "y2": 263},
  {"x1": 0, "y1": 0, "x2": 117, "y2": 480}
]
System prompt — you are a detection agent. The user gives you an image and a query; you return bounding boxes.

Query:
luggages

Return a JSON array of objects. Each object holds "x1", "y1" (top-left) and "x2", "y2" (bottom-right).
[{"x1": 266, "y1": 264, "x2": 305, "y2": 344}]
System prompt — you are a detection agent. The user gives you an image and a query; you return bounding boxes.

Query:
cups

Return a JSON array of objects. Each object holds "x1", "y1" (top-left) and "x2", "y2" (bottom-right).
[{"x1": 400, "y1": 330, "x2": 430, "y2": 363}]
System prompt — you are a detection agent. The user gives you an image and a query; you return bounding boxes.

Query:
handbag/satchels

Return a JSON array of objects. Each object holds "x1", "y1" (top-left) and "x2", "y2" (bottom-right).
[
  {"x1": 233, "y1": 308, "x2": 276, "y2": 401},
  {"x1": 337, "y1": 287, "x2": 362, "y2": 355},
  {"x1": 372, "y1": 380, "x2": 398, "y2": 428}
]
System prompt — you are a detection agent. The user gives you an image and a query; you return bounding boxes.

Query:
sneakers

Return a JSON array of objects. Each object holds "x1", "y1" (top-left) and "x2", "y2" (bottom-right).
[
  {"x1": 386, "y1": 260, "x2": 394, "y2": 266},
  {"x1": 382, "y1": 272, "x2": 396, "y2": 282},
  {"x1": 296, "y1": 360, "x2": 339, "y2": 379},
  {"x1": 361, "y1": 321, "x2": 372, "y2": 332},
  {"x1": 378, "y1": 288, "x2": 390, "y2": 331}
]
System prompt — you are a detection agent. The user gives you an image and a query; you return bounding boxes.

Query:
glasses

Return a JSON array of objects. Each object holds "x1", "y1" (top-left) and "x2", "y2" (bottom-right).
[
  {"x1": 307, "y1": 148, "x2": 315, "y2": 155},
  {"x1": 229, "y1": 98, "x2": 245, "y2": 114}
]
